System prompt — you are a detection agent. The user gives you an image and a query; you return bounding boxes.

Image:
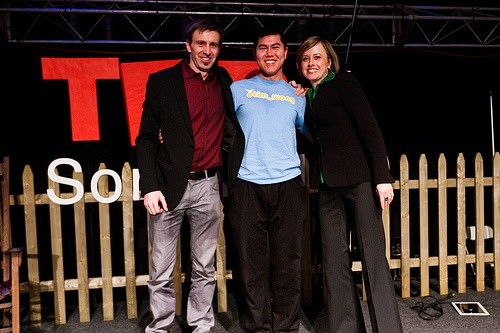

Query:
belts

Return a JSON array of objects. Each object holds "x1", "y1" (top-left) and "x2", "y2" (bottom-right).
[{"x1": 189, "y1": 168, "x2": 215, "y2": 181}]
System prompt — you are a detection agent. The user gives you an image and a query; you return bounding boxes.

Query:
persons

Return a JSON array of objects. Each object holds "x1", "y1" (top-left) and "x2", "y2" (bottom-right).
[
  {"x1": 224, "y1": 32, "x2": 315, "y2": 333},
  {"x1": 132, "y1": 22, "x2": 245, "y2": 333},
  {"x1": 292, "y1": 30, "x2": 406, "y2": 333}
]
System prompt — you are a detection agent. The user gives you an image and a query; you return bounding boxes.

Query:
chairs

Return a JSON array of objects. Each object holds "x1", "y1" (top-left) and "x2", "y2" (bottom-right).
[{"x1": 0, "y1": 156, "x2": 22, "y2": 333}]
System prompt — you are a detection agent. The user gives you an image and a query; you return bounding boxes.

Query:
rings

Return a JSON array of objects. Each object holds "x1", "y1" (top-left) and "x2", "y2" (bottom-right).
[{"x1": 383, "y1": 196, "x2": 389, "y2": 201}]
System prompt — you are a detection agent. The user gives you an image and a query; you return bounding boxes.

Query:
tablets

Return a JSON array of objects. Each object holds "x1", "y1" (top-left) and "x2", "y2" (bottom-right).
[{"x1": 451, "y1": 301, "x2": 490, "y2": 316}]
